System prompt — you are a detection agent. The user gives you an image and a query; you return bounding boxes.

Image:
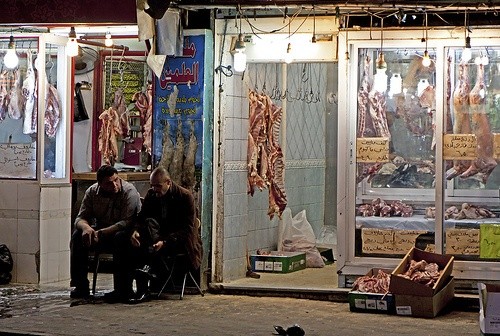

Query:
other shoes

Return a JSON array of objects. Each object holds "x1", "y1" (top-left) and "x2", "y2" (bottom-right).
[
  {"x1": 70, "y1": 286, "x2": 90, "y2": 298},
  {"x1": 104, "y1": 288, "x2": 135, "y2": 301},
  {"x1": 126, "y1": 290, "x2": 152, "y2": 303}
]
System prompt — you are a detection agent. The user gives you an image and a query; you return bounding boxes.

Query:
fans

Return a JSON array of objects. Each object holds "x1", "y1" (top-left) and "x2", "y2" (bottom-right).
[{"x1": 75, "y1": 46, "x2": 100, "y2": 75}]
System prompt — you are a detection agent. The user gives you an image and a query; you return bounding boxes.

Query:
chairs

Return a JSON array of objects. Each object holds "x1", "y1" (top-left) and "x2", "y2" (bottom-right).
[{"x1": 71, "y1": 217, "x2": 205, "y2": 300}]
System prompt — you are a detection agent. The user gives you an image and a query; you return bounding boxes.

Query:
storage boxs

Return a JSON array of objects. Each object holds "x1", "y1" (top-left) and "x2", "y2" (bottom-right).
[
  {"x1": 478, "y1": 283, "x2": 500, "y2": 334},
  {"x1": 249, "y1": 250, "x2": 306, "y2": 273},
  {"x1": 316, "y1": 246, "x2": 334, "y2": 265},
  {"x1": 388, "y1": 247, "x2": 454, "y2": 297},
  {"x1": 395, "y1": 276, "x2": 453, "y2": 316},
  {"x1": 347, "y1": 265, "x2": 395, "y2": 313}
]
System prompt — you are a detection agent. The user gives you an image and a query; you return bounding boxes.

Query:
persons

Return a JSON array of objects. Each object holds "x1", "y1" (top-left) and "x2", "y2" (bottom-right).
[
  {"x1": 108, "y1": 167, "x2": 202, "y2": 303},
  {"x1": 70, "y1": 165, "x2": 142, "y2": 300}
]
